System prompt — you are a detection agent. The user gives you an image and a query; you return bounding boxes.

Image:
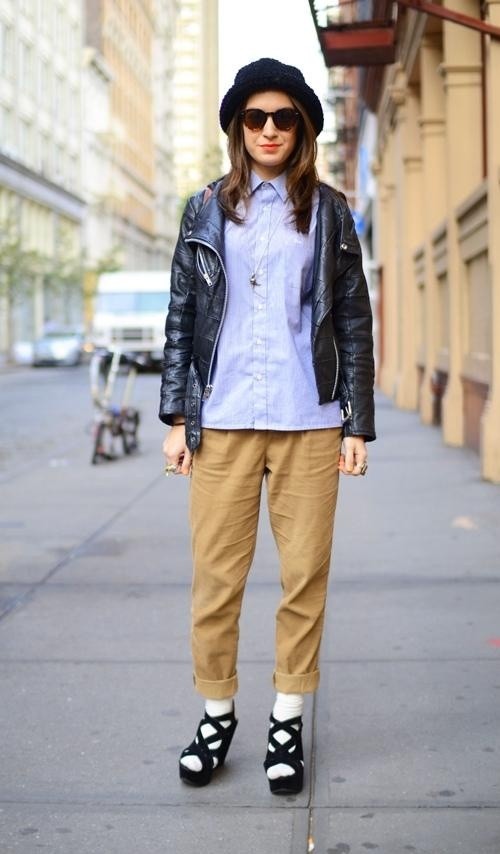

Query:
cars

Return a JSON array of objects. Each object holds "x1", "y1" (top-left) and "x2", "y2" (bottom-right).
[{"x1": 10, "y1": 325, "x2": 86, "y2": 369}]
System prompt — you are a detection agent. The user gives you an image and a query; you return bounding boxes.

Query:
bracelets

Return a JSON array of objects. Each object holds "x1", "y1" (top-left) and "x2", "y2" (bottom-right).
[{"x1": 167, "y1": 419, "x2": 188, "y2": 431}]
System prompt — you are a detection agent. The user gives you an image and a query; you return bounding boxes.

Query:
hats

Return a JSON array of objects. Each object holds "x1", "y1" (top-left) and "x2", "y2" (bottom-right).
[{"x1": 219, "y1": 57, "x2": 324, "y2": 139}]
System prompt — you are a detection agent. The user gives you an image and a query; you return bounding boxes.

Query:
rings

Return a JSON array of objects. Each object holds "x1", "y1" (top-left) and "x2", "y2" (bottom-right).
[
  {"x1": 356, "y1": 462, "x2": 371, "y2": 478},
  {"x1": 162, "y1": 464, "x2": 184, "y2": 478}
]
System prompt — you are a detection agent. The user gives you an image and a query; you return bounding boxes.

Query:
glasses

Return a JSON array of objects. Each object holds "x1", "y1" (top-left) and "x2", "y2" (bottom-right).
[{"x1": 237, "y1": 106, "x2": 301, "y2": 132}]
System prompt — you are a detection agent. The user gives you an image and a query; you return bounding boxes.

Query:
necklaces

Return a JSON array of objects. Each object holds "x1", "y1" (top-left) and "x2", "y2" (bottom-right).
[{"x1": 237, "y1": 176, "x2": 294, "y2": 288}]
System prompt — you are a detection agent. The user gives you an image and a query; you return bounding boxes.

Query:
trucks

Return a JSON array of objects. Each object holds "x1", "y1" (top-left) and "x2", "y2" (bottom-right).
[{"x1": 93, "y1": 269, "x2": 171, "y2": 370}]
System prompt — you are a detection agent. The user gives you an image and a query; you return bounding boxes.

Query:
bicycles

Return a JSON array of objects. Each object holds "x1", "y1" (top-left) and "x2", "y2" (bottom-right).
[{"x1": 86, "y1": 339, "x2": 154, "y2": 463}]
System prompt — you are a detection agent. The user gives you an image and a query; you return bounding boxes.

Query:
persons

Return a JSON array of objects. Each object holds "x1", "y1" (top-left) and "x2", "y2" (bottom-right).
[{"x1": 159, "y1": 57, "x2": 377, "y2": 796}]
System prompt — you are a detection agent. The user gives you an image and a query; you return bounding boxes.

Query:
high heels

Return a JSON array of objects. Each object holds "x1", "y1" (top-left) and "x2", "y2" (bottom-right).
[
  {"x1": 264, "y1": 711, "x2": 305, "y2": 794},
  {"x1": 179, "y1": 698, "x2": 238, "y2": 785}
]
